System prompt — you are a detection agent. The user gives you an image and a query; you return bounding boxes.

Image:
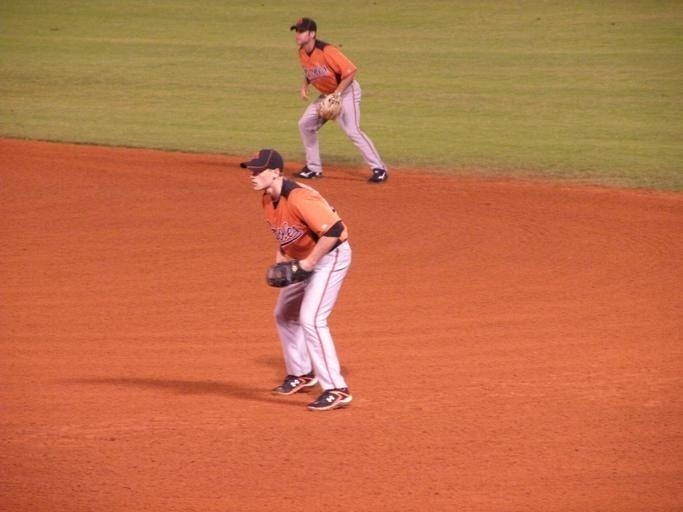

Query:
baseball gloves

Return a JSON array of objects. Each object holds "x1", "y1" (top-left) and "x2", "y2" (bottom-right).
[
  {"x1": 266, "y1": 260, "x2": 314, "y2": 287},
  {"x1": 319, "y1": 91, "x2": 343, "y2": 119}
]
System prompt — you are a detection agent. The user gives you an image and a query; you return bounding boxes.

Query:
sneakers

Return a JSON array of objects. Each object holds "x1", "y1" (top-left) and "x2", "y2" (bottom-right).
[
  {"x1": 307, "y1": 387, "x2": 352, "y2": 411},
  {"x1": 271, "y1": 371, "x2": 318, "y2": 395},
  {"x1": 368, "y1": 168, "x2": 389, "y2": 184},
  {"x1": 293, "y1": 166, "x2": 322, "y2": 179}
]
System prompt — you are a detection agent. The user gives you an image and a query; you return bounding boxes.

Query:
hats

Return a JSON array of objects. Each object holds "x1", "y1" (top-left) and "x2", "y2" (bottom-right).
[
  {"x1": 240, "y1": 149, "x2": 283, "y2": 172},
  {"x1": 290, "y1": 17, "x2": 316, "y2": 32}
]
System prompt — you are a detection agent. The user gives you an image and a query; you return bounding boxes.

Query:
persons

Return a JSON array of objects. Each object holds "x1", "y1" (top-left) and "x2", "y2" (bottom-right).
[
  {"x1": 239, "y1": 147, "x2": 353, "y2": 411},
  {"x1": 289, "y1": 17, "x2": 387, "y2": 183}
]
